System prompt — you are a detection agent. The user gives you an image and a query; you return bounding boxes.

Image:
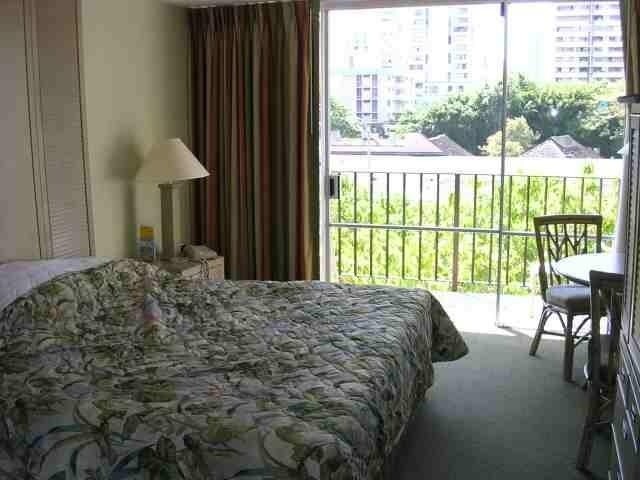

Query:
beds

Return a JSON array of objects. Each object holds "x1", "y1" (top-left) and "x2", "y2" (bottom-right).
[{"x1": 0, "y1": 258, "x2": 468, "y2": 480}]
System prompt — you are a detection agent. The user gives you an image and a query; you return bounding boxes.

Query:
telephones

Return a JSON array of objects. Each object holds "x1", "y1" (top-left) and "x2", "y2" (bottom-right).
[{"x1": 183, "y1": 245, "x2": 218, "y2": 279}]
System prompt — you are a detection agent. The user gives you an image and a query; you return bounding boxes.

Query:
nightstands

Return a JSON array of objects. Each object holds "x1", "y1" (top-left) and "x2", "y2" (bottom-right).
[{"x1": 183, "y1": 256, "x2": 224, "y2": 283}]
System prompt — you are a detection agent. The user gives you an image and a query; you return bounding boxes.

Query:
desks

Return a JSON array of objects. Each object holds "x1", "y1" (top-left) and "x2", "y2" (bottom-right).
[{"x1": 556, "y1": 253, "x2": 618, "y2": 388}]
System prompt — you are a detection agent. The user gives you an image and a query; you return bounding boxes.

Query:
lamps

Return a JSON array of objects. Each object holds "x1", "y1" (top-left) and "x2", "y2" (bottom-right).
[{"x1": 135, "y1": 138, "x2": 210, "y2": 265}]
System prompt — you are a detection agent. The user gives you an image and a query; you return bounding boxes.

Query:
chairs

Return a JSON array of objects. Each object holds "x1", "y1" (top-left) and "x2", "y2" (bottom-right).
[
  {"x1": 529, "y1": 215, "x2": 603, "y2": 382},
  {"x1": 575, "y1": 270, "x2": 626, "y2": 473}
]
